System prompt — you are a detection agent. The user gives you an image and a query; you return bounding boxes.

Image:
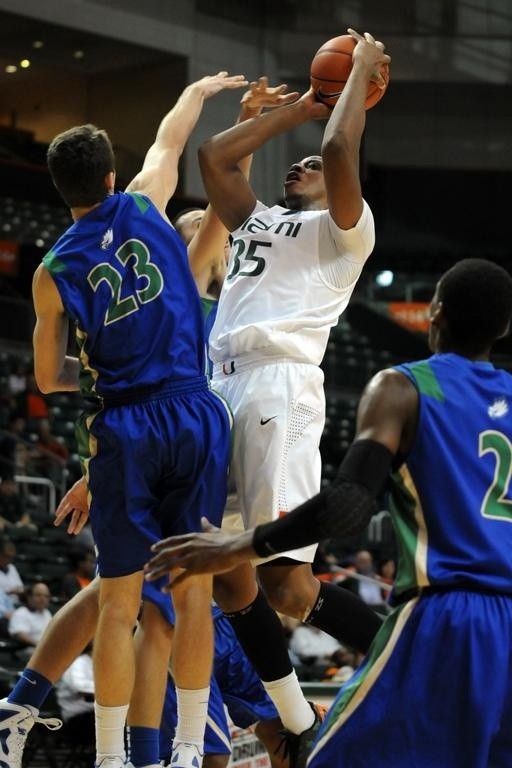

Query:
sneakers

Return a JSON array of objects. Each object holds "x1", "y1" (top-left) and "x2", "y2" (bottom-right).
[
  {"x1": 0, "y1": 695, "x2": 41, "y2": 768},
  {"x1": 277, "y1": 700, "x2": 330, "y2": 768},
  {"x1": 95, "y1": 740, "x2": 207, "y2": 768}
]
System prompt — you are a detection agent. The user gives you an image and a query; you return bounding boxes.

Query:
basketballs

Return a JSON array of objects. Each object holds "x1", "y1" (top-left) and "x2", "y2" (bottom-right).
[{"x1": 310, "y1": 35, "x2": 390, "y2": 112}]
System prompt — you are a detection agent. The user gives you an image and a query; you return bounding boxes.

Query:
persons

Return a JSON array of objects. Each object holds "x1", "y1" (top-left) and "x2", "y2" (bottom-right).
[
  {"x1": 143, "y1": 256, "x2": 512, "y2": 765},
  {"x1": 2, "y1": 21, "x2": 396, "y2": 768}
]
